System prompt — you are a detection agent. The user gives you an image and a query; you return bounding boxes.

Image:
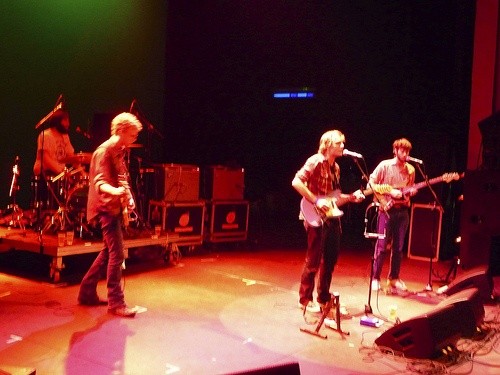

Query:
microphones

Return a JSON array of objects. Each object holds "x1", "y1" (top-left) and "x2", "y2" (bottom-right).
[
  {"x1": 342, "y1": 149, "x2": 362, "y2": 159},
  {"x1": 406, "y1": 156, "x2": 422, "y2": 164},
  {"x1": 129, "y1": 99, "x2": 135, "y2": 113}
]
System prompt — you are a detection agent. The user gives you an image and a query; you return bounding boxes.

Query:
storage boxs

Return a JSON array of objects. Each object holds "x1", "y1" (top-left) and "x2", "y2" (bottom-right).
[
  {"x1": 147, "y1": 199, "x2": 250, "y2": 242},
  {"x1": 407, "y1": 203, "x2": 452, "y2": 262}
]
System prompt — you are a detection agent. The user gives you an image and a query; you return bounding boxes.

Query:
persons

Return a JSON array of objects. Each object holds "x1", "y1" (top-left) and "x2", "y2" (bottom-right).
[
  {"x1": 367, "y1": 139, "x2": 417, "y2": 291},
  {"x1": 77, "y1": 113, "x2": 142, "y2": 317},
  {"x1": 291, "y1": 130, "x2": 365, "y2": 312},
  {"x1": 34, "y1": 109, "x2": 86, "y2": 184}
]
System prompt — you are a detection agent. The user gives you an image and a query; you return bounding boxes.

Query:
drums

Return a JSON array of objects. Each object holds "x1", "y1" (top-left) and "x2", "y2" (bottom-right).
[{"x1": 64, "y1": 181, "x2": 90, "y2": 230}]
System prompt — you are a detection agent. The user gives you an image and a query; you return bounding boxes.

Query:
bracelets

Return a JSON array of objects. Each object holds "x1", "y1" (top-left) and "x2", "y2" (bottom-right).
[{"x1": 315, "y1": 198, "x2": 319, "y2": 204}]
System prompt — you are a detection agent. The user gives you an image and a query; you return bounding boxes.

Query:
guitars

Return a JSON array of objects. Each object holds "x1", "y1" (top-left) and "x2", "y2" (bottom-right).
[
  {"x1": 117, "y1": 167, "x2": 130, "y2": 227},
  {"x1": 298, "y1": 185, "x2": 391, "y2": 229},
  {"x1": 383, "y1": 171, "x2": 461, "y2": 212}
]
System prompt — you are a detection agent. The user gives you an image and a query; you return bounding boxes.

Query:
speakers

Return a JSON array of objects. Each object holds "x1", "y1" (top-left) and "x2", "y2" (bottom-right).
[
  {"x1": 150, "y1": 166, "x2": 199, "y2": 203},
  {"x1": 436, "y1": 289, "x2": 484, "y2": 333},
  {"x1": 443, "y1": 265, "x2": 494, "y2": 301},
  {"x1": 459, "y1": 173, "x2": 500, "y2": 267},
  {"x1": 374, "y1": 299, "x2": 472, "y2": 358},
  {"x1": 200, "y1": 167, "x2": 244, "y2": 201},
  {"x1": 408, "y1": 203, "x2": 451, "y2": 262}
]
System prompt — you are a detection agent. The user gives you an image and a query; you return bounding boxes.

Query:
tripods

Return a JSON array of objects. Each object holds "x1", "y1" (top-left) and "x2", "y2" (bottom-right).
[
  {"x1": 350, "y1": 156, "x2": 389, "y2": 322},
  {"x1": 401, "y1": 163, "x2": 442, "y2": 299}
]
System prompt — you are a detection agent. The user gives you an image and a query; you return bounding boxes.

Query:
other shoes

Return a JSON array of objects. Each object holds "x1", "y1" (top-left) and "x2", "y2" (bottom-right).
[
  {"x1": 371, "y1": 280, "x2": 381, "y2": 290},
  {"x1": 386, "y1": 278, "x2": 407, "y2": 290},
  {"x1": 298, "y1": 301, "x2": 320, "y2": 312},
  {"x1": 320, "y1": 301, "x2": 344, "y2": 314},
  {"x1": 95, "y1": 299, "x2": 109, "y2": 306},
  {"x1": 107, "y1": 306, "x2": 137, "y2": 318}
]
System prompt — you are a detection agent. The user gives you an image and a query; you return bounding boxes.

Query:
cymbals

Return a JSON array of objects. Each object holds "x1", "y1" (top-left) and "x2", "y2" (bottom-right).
[{"x1": 61, "y1": 153, "x2": 92, "y2": 163}]
